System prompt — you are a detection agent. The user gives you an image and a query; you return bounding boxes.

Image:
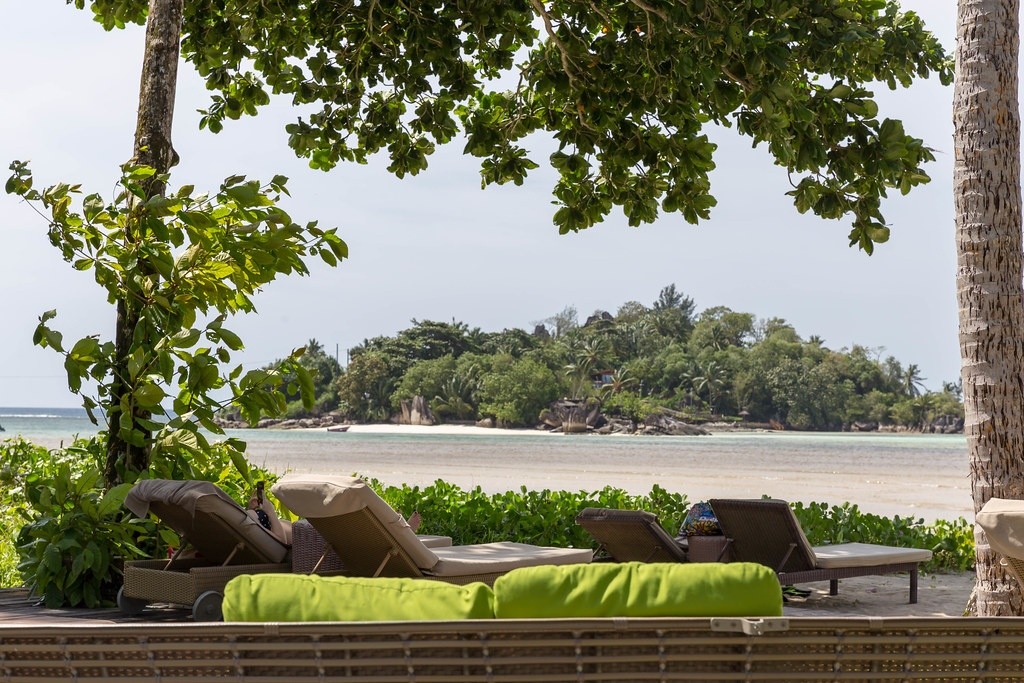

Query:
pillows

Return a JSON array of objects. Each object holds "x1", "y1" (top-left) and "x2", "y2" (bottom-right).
[
  {"x1": 492, "y1": 563, "x2": 783, "y2": 618},
  {"x1": 221, "y1": 574, "x2": 494, "y2": 621}
]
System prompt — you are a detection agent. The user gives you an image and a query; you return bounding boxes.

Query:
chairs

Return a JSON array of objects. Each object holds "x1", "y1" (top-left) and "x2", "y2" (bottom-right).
[
  {"x1": 576, "y1": 508, "x2": 689, "y2": 563},
  {"x1": 118, "y1": 479, "x2": 453, "y2": 621},
  {"x1": 270, "y1": 470, "x2": 591, "y2": 589},
  {"x1": 709, "y1": 498, "x2": 932, "y2": 604}
]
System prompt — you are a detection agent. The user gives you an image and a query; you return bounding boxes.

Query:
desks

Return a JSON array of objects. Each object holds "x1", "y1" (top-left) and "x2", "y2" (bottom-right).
[{"x1": 687, "y1": 535, "x2": 729, "y2": 562}]
